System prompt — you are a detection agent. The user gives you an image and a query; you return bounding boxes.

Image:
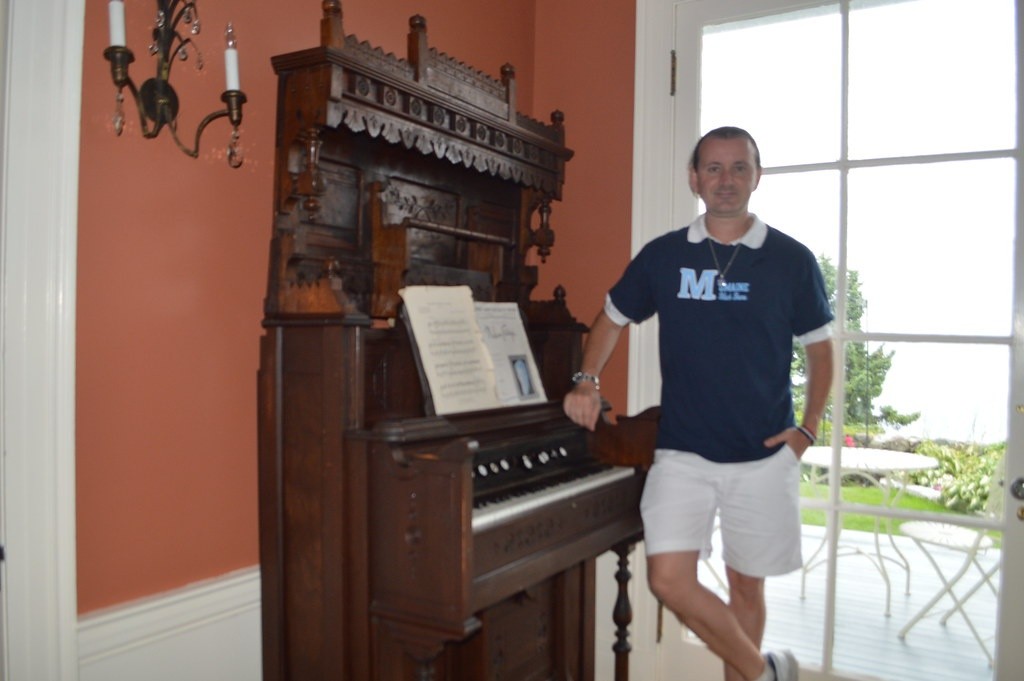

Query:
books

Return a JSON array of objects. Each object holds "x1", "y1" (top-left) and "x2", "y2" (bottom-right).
[{"x1": 398, "y1": 285, "x2": 548, "y2": 415}]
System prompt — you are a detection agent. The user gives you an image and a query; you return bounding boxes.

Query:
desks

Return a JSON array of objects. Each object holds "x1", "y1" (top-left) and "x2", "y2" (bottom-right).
[{"x1": 797, "y1": 445, "x2": 938, "y2": 617}]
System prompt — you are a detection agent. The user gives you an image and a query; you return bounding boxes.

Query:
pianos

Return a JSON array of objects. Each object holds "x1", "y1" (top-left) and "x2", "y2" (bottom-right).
[{"x1": 253, "y1": 281, "x2": 664, "y2": 680}]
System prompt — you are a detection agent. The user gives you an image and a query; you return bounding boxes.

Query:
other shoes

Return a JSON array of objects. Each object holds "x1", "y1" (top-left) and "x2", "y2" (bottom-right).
[{"x1": 762, "y1": 648, "x2": 799, "y2": 681}]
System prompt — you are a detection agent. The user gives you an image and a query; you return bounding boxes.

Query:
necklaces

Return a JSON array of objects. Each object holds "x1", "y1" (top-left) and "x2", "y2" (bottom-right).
[{"x1": 708, "y1": 219, "x2": 753, "y2": 286}]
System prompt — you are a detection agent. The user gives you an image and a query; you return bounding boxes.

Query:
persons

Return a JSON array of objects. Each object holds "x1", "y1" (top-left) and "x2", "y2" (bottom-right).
[{"x1": 564, "y1": 127, "x2": 833, "y2": 681}]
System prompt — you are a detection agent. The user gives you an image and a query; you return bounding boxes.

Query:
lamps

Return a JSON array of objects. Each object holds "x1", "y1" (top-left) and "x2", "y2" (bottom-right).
[{"x1": 103, "y1": 0, "x2": 247, "y2": 167}]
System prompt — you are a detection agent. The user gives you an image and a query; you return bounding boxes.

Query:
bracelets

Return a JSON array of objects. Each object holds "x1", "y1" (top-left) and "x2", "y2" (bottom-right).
[
  {"x1": 572, "y1": 372, "x2": 600, "y2": 390},
  {"x1": 797, "y1": 425, "x2": 816, "y2": 445}
]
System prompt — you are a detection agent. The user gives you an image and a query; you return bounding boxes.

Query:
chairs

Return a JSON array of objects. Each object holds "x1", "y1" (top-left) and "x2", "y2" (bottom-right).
[{"x1": 899, "y1": 453, "x2": 1005, "y2": 666}]
